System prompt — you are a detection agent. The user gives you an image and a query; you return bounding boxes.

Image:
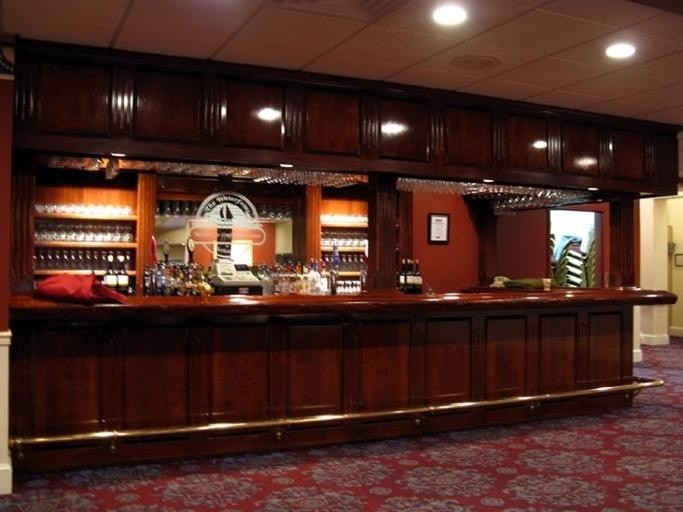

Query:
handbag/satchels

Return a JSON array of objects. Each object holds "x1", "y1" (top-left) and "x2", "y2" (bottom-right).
[{"x1": 37, "y1": 275, "x2": 104, "y2": 303}]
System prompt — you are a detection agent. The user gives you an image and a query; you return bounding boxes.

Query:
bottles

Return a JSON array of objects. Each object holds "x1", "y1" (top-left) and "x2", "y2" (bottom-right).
[
  {"x1": 103, "y1": 260, "x2": 130, "y2": 294},
  {"x1": 294, "y1": 246, "x2": 364, "y2": 274},
  {"x1": 397, "y1": 258, "x2": 423, "y2": 294},
  {"x1": 263, "y1": 271, "x2": 308, "y2": 296}
]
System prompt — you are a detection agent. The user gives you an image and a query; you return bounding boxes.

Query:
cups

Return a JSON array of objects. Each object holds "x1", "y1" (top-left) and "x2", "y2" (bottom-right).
[{"x1": 543, "y1": 278, "x2": 551, "y2": 291}]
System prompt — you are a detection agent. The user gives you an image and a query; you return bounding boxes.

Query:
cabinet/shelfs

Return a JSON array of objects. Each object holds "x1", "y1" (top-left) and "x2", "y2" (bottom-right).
[
  {"x1": 18, "y1": 323, "x2": 104, "y2": 447},
  {"x1": 131, "y1": 68, "x2": 203, "y2": 143},
  {"x1": 484, "y1": 315, "x2": 528, "y2": 410},
  {"x1": 35, "y1": 58, "x2": 107, "y2": 134},
  {"x1": 31, "y1": 174, "x2": 138, "y2": 289},
  {"x1": 303, "y1": 91, "x2": 364, "y2": 156},
  {"x1": 506, "y1": 114, "x2": 548, "y2": 174},
  {"x1": 426, "y1": 320, "x2": 471, "y2": 415},
  {"x1": 227, "y1": 78, "x2": 285, "y2": 150},
  {"x1": 650, "y1": 135, "x2": 680, "y2": 187},
  {"x1": 359, "y1": 320, "x2": 414, "y2": 426},
  {"x1": 211, "y1": 326, "x2": 274, "y2": 438},
  {"x1": 118, "y1": 326, "x2": 196, "y2": 442},
  {"x1": 541, "y1": 315, "x2": 578, "y2": 402},
  {"x1": 288, "y1": 325, "x2": 345, "y2": 429},
  {"x1": 590, "y1": 310, "x2": 629, "y2": 391},
  {"x1": 322, "y1": 196, "x2": 378, "y2": 292},
  {"x1": 609, "y1": 127, "x2": 645, "y2": 182},
  {"x1": 444, "y1": 106, "x2": 490, "y2": 168},
  {"x1": 379, "y1": 100, "x2": 431, "y2": 164},
  {"x1": 564, "y1": 123, "x2": 601, "y2": 173}
]
